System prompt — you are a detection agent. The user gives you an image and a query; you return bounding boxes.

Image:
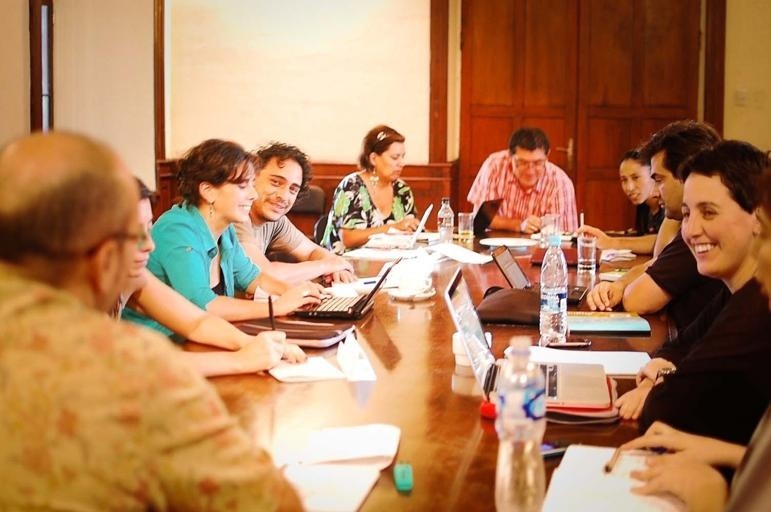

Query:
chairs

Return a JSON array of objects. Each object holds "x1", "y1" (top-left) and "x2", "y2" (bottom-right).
[
  {"x1": 313, "y1": 214, "x2": 331, "y2": 245},
  {"x1": 264, "y1": 185, "x2": 327, "y2": 262}
]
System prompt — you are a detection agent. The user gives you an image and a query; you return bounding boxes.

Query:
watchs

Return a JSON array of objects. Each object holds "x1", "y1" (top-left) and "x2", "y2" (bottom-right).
[{"x1": 659, "y1": 366, "x2": 678, "y2": 378}]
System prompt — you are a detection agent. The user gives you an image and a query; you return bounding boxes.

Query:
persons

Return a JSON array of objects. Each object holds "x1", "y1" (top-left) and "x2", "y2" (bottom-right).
[
  {"x1": 468, "y1": 127, "x2": 580, "y2": 236},
  {"x1": 231, "y1": 141, "x2": 362, "y2": 284},
  {"x1": 122, "y1": 138, "x2": 336, "y2": 346},
  {"x1": 106, "y1": 174, "x2": 308, "y2": 379},
  {"x1": 578, "y1": 148, "x2": 668, "y2": 253},
  {"x1": 0, "y1": 125, "x2": 309, "y2": 512},
  {"x1": 318, "y1": 123, "x2": 434, "y2": 255},
  {"x1": 586, "y1": 120, "x2": 726, "y2": 328}
]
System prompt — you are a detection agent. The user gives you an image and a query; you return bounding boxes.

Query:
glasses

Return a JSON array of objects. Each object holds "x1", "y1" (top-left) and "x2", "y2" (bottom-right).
[{"x1": 512, "y1": 158, "x2": 549, "y2": 171}]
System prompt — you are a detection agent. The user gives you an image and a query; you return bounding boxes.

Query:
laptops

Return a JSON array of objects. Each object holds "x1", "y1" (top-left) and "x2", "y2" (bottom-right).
[
  {"x1": 446, "y1": 269, "x2": 618, "y2": 418},
  {"x1": 453, "y1": 199, "x2": 502, "y2": 235},
  {"x1": 365, "y1": 203, "x2": 434, "y2": 250},
  {"x1": 530, "y1": 247, "x2": 600, "y2": 268},
  {"x1": 294, "y1": 256, "x2": 402, "y2": 321},
  {"x1": 492, "y1": 246, "x2": 588, "y2": 306}
]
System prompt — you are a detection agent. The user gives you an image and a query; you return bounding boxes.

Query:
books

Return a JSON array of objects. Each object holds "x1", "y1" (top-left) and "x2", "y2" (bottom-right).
[
  {"x1": 568, "y1": 310, "x2": 652, "y2": 334},
  {"x1": 236, "y1": 315, "x2": 356, "y2": 350}
]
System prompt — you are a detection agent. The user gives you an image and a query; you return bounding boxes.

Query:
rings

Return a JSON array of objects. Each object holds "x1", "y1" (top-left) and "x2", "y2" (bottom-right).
[
  {"x1": 304, "y1": 288, "x2": 308, "y2": 297},
  {"x1": 531, "y1": 222, "x2": 534, "y2": 230},
  {"x1": 406, "y1": 222, "x2": 410, "y2": 227}
]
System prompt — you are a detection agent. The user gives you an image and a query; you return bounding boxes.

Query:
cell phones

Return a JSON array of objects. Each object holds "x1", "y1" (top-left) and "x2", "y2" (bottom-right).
[{"x1": 542, "y1": 439, "x2": 576, "y2": 461}]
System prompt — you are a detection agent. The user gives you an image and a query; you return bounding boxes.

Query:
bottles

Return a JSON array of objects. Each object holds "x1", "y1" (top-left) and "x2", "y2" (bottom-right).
[
  {"x1": 537, "y1": 231, "x2": 569, "y2": 343},
  {"x1": 435, "y1": 196, "x2": 457, "y2": 255},
  {"x1": 492, "y1": 332, "x2": 548, "y2": 512}
]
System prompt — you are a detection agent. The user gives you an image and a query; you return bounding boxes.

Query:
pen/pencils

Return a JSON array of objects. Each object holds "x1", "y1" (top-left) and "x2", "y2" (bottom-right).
[
  {"x1": 580, "y1": 209, "x2": 584, "y2": 237},
  {"x1": 605, "y1": 448, "x2": 621, "y2": 472},
  {"x1": 269, "y1": 296, "x2": 275, "y2": 331}
]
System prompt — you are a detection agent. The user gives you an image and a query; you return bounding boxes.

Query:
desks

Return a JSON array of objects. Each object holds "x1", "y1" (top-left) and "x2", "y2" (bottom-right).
[{"x1": 169, "y1": 228, "x2": 682, "y2": 512}]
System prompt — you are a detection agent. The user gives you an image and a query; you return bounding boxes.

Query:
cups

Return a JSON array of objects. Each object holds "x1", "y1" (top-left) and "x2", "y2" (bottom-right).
[
  {"x1": 577, "y1": 235, "x2": 597, "y2": 274},
  {"x1": 457, "y1": 213, "x2": 477, "y2": 243},
  {"x1": 574, "y1": 273, "x2": 596, "y2": 293}
]
